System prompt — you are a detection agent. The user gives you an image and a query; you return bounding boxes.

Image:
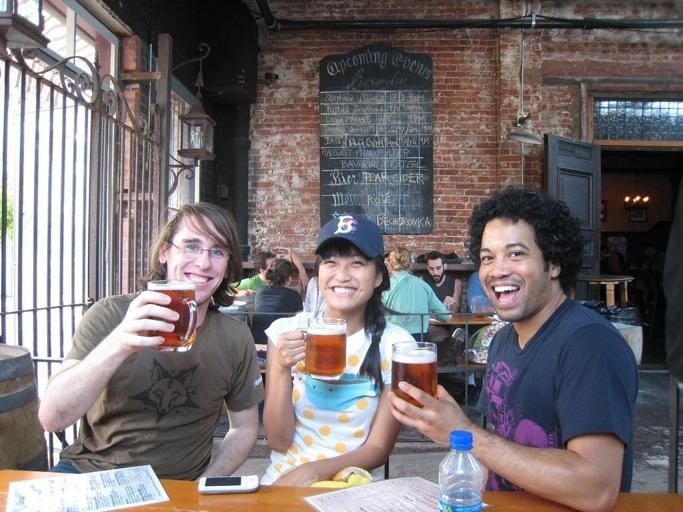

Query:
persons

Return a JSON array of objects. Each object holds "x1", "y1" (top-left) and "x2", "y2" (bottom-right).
[
  {"x1": 390, "y1": 187, "x2": 638, "y2": 512},
  {"x1": 261, "y1": 214, "x2": 417, "y2": 488},
  {"x1": 37, "y1": 201, "x2": 264, "y2": 479},
  {"x1": 228, "y1": 246, "x2": 493, "y2": 368}
]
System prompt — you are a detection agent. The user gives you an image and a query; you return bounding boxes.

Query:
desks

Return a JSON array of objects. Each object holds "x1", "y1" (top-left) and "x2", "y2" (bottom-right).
[
  {"x1": 2, "y1": 466, "x2": 683, "y2": 512},
  {"x1": 432, "y1": 309, "x2": 496, "y2": 332}
]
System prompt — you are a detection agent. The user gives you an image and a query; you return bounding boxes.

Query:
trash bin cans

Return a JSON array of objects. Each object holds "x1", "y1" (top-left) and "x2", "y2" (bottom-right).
[{"x1": 570, "y1": 274, "x2": 643, "y2": 370}]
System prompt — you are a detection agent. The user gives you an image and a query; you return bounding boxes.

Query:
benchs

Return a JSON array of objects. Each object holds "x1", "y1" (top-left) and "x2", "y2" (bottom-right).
[{"x1": 216, "y1": 360, "x2": 494, "y2": 419}]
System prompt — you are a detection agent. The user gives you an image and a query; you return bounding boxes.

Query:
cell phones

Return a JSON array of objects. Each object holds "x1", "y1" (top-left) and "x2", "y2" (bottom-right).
[{"x1": 198, "y1": 475, "x2": 259, "y2": 494}]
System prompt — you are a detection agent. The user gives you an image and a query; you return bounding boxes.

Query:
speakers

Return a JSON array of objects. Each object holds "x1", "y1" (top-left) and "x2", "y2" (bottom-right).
[{"x1": 207, "y1": 0, "x2": 257, "y2": 105}]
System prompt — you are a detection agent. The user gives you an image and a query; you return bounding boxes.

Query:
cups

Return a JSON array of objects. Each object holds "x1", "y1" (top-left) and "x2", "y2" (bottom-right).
[
  {"x1": 294, "y1": 317, "x2": 347, "y2": 380},
  {"x1": 391, "y1": 339, "x2": 438, "y2": 416},
  {"x1": 147, "y1": 278, "x2": 197, "y2": 352}
]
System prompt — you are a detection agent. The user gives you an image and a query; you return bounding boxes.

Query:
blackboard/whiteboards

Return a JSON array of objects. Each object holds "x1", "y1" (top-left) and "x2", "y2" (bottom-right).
[{"x1": 319, "y1": 44, "x2": 433, "y2": 235}]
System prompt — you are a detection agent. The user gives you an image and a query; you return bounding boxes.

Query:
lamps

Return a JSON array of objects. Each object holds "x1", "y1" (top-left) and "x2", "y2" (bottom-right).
[
  {"x1": 505, "y1": 111, "x2": 544, "y2": 147},
  {"x1": 177, "y1": 65, "x2": 217, "y2": 162}
]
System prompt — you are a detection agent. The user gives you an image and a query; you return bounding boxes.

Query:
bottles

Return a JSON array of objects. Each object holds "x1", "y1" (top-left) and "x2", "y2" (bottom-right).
[{"x1": 437, "y1": 430, "x2": 483, "y2": 512}]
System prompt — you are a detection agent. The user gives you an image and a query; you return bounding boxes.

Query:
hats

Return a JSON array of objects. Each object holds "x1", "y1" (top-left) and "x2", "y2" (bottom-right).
[{"x1": 315, "y1": 214, "x2": 384, "y2": 261}]
